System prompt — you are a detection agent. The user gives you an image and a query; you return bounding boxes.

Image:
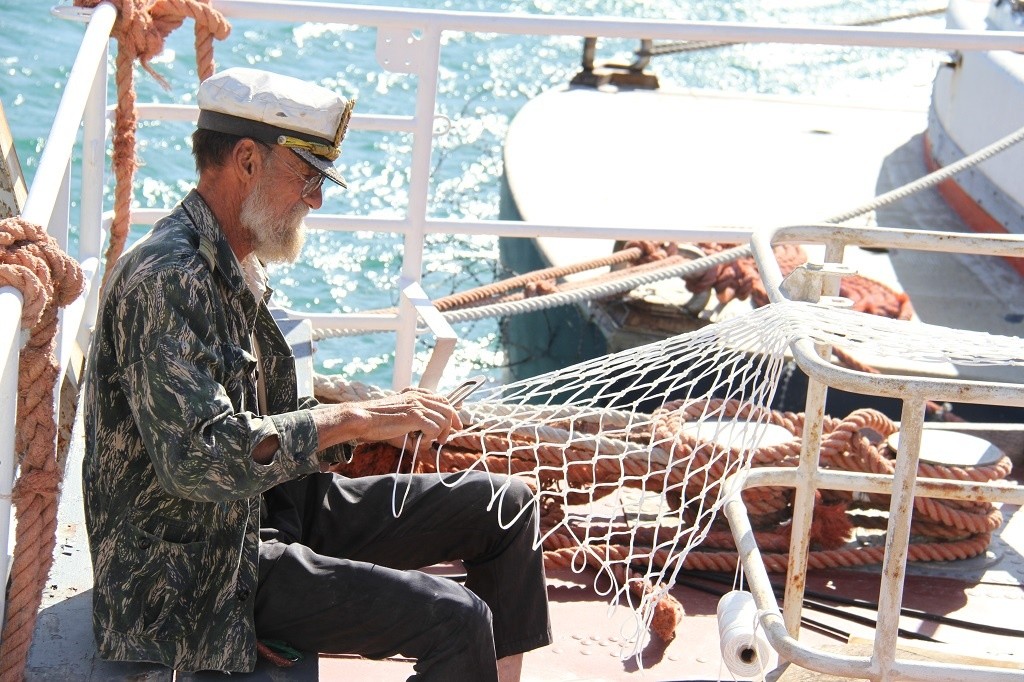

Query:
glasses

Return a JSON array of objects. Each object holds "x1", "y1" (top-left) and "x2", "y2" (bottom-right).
[{"x1": 255, "y1": 137, "x2": 327, "y2": 200}]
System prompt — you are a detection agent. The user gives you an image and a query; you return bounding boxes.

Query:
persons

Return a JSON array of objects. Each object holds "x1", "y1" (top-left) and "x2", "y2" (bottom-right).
[{"x1": 81, "y1": 67, "x2": 553, "y2": 682}]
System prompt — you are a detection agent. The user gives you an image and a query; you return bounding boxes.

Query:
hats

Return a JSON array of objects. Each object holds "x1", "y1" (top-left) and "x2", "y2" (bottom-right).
[{"x1": 196, "y1": 68, "x2": 353, "y2": 190}]
